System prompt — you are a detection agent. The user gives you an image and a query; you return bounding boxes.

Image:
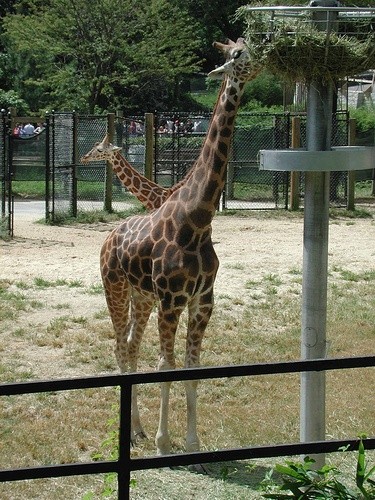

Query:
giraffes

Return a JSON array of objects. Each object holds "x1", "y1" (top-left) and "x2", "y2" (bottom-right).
[
  {"x1": 99, "y1": 37, "x2": 265, "y2": 471},
  {"x1": 79, "y1": 133, "x2": 172, "y2": 213}
]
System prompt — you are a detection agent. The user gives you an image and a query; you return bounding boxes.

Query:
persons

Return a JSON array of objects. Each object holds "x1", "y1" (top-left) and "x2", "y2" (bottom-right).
[
  {"x1": 119, "y1": 117, "x2": 203, "y2": 138},
  {"x1": 8, "y1": 122, "x2": 47, "y2": 138}
]
